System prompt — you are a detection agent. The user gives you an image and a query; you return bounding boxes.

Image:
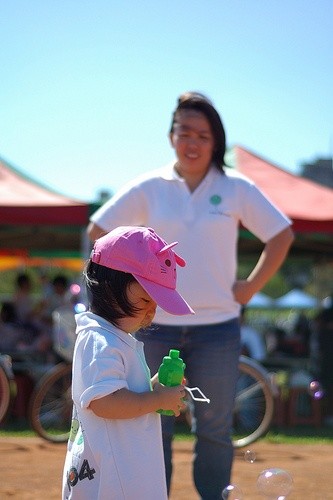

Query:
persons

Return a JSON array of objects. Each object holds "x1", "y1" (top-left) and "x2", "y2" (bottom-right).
[
  {"x1": 84, "y1": 91, "x2": 295, "y2": 500},
  {"x1": 239, "y1": 307, "x2": 333, "y2": 428},
  {"x1": 0, "y1": 273, "x2": 76, "y2": 423},
  {"x1": 62, "y1": 226, "x2": 194, "y2": 500}
]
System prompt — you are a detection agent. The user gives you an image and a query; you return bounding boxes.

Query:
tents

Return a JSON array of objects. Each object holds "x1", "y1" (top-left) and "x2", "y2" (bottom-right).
[
  {"x1": 1, "y1": 157, "x2": 88, "y2": 313},
  {"x1": 223, "y1": 143, "x2": 333, "y2": 234}
]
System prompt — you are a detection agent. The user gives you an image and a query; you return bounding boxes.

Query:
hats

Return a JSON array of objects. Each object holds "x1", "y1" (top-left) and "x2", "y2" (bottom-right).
[{"x1": 91, "y1": 226, "x2": 196, "y2": 315}]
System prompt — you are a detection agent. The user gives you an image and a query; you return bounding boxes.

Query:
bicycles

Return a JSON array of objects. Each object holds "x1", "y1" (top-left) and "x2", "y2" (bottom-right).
[{"x1": 26, "y1": 303, "x2": 278, "y2": 450}]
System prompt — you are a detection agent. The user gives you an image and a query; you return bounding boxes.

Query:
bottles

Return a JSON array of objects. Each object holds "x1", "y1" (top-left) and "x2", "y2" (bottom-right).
[{"x1": 153, "y1": 350, "x2": 186, "y2": 416}]
